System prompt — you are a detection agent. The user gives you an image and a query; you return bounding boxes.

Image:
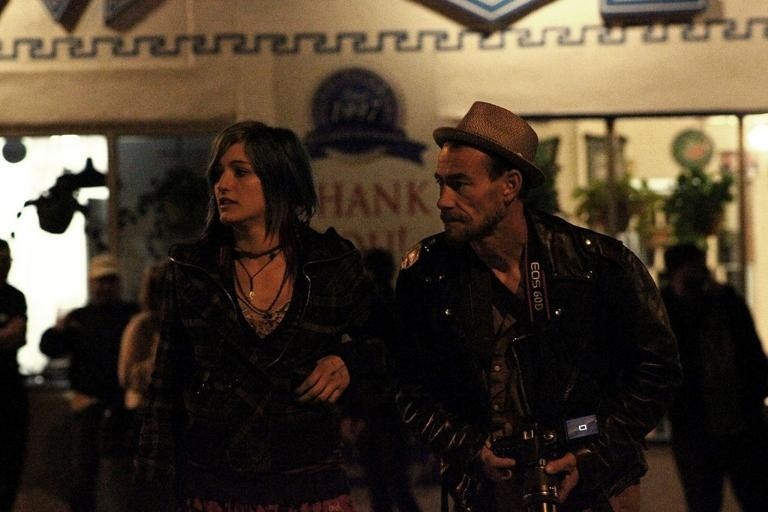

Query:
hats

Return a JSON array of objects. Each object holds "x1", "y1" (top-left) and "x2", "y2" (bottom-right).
[{"x1": 432, "y1": 101, "x2": 546, "y2": 190}]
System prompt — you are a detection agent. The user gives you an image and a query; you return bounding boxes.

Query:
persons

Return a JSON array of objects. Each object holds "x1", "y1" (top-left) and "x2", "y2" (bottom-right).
[
  {"x1": 1, "y1": 240, "x2": 28, "y2": 511},
  {"x1": 389, "y1": 102, "x2": 684, "y2": 511},
  {"x1": 658, "y1": 241, "x2": 767, "y2": 511},
  {"x1": 115, "y1": 261, "x2": 165, "y2": 422},
  {"x1": 61, "y1": 253, "x2": 140, "y2": 512},
  {"x1": 129, "y1": 119, "x2": 389, "y2": 511}
]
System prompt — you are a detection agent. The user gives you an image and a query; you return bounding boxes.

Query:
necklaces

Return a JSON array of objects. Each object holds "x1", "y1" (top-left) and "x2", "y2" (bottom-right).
[{"x1": 229, "y1": 249, "x2": 283, "y2": 299}]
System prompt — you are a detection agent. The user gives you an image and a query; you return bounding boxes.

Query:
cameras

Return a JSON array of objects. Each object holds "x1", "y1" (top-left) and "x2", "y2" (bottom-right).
[{"x1": 491, "y1": 420, "x2": 566, "y2": 512}]
